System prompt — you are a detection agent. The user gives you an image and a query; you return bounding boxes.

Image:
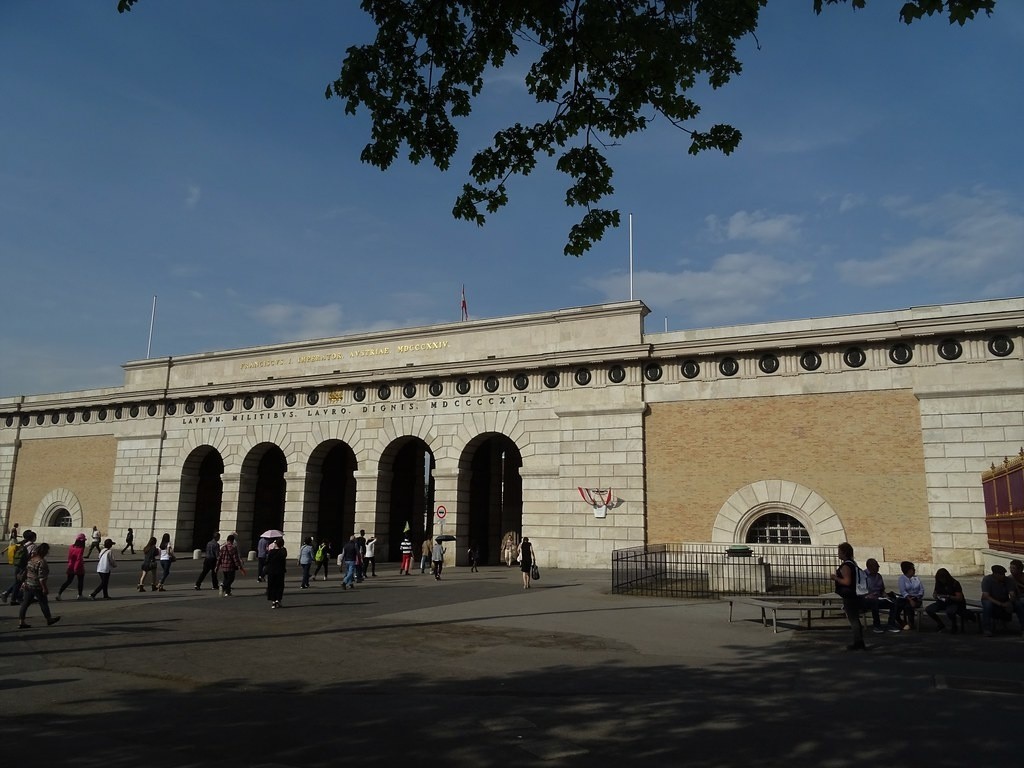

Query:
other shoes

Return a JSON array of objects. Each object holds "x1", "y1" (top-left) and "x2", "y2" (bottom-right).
[
  {"x1": 0, "y1": 568, "x2": 529, "y2": 629},
  {"x1": 848, "y1": 621, "x2": 992, "y2": 650}
]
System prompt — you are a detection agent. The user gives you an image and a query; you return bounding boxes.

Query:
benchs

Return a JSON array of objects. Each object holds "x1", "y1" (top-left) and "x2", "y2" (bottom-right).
[{"x1": 720, "y1": 592, "x2": 1012, "y2": 634}]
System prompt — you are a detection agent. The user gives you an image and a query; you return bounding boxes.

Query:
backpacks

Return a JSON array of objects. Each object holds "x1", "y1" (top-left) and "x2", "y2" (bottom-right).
[
  {"x1": 844, "y1": 561, "x2": 869, "y2": 595},
  {"x1": 314, "y1": 545, "x2": 325, "y2": 561},
  {"x1": 13, "y1": 543, "x2": 33, "y2": 567},
  {"x1": 7, "y1": 543, "x2": 21, "y2": 564}
]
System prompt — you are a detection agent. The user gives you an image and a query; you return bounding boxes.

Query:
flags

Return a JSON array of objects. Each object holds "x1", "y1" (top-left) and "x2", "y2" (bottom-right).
[{"x1": 461, "y1": 293, "x2": 468, "y2": 320}]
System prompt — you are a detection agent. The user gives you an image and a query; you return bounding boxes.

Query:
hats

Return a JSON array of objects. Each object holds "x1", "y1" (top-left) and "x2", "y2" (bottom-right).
[
  {"x1": 404, "y1": 534, "x2": 408, "y2": 538},
  {"x1": 76, "y1": 534, "x2": 87, "y2": 540},
  {"x1": 992, "y1": 565, "x2": 1007, "y2": 573},
  {"x1": 105, "y1": 539, "x2": 115, "y2": 544}
]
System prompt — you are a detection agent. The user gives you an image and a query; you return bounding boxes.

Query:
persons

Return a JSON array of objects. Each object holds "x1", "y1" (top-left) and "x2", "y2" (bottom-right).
[
  {"x1": 0, "y1": 523, "x2": 61, "y2": 629},
  {"x1": 980, "y1": 560, "x2": 1024, "y2": 636},
  {"x1": 898, "y1": 561, "x2": 924, "y2": 630},
  {"x1": 432, "y1": 540, "x2": 446, "y2": 580},
  {"x1": 400, "y1": 536, "x2": 414, "y2": 575},
  {"x1": 84, "y1": 525, "x2": 175, "y2": 599},
  {"x1": 517, "y1": 537, "x2": 534, "y2": 589},
  {"x1": 55, "y1": 533, "x2": 88, "y2": 600},
  {"x1": 420, "y1": 535, "x2": 432, "y2": 573},
  {"x1": 196, "y1": 533, "x2": 247, "y2": 597},
  {"x1": 830, "y1": 541, "x2": 865, "y2": 650},
  {"x1": 297, "y1": 530, "x2": 377, "y2": 589},
  {"x1": 862, "y1": 558, "x2": 902, "y2": 633},
  {"x1": 256, "y1": 538, "x2": 287, "y2": 608},
  {"x1": 502, "y1": 535, "x2": 514, "y2": 567},
  {"x1": 470, "y1": 550, "x2": 479, "y2": 572},
  {"x1": 926, "y1": 568, "x2": 966, "y2": 635}
]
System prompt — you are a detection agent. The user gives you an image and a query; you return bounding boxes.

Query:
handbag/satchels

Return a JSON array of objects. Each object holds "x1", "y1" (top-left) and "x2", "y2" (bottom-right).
[
  {"x1": 410, "y1": 557, "x2": 415, "y2": 569},
  {"x1": 431, "y1": 562, "x2": 435, "y2": 569},
  {"x1": 150, "y1": 562, "x2": 157, "y2": 569},
  {"x1": 420, "y1": 557, "x2": 424, "y2": 568},
  {"x1": 336, "y1": 554, "x2": 344, "y2": 567},
  {"x1": 233, "y1": 562, "x2": 238, "y2": 569},
  {"x1": 168, "y1": 547, "x2": 177, "y2": 562},
  {"x1": 532, "y1": 561, "x2": 540, "y2": 580},
  {"x1": 888, "y1": 591, "x2": 902, "y2": 608}
]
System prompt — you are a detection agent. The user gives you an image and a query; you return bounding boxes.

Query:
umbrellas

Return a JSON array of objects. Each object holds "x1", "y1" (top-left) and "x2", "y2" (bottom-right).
[
  {"x1": 435, "y1": 535, "x2": 456, "y2": 541},
  {"x1": 260, "y1": 530, "x2": 285, "y2": 537}
]
